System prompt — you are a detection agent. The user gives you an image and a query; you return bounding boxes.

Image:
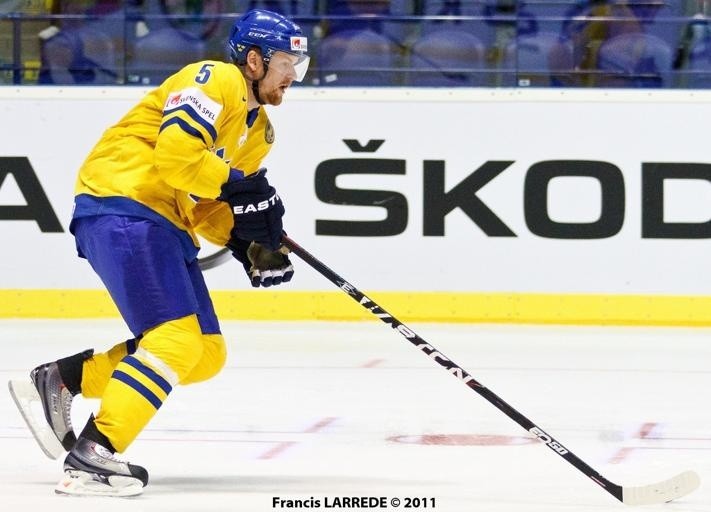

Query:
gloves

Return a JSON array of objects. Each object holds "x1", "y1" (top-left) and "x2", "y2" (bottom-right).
[
  {"x1": 216, "y1": 166, "x2": 285, "y2": 241},
  {"x1": 225, "y1": 226, "x2": 294, "y2": 286}
]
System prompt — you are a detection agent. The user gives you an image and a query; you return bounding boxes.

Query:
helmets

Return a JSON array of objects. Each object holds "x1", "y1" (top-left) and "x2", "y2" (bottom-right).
[{"x1": 228, "y1": 9, "x2": 310, "y2": 82}]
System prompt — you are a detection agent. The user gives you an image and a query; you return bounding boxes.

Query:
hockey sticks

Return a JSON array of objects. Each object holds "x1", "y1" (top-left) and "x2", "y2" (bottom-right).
[{"x1": 282, "y1": 236, "x2": 702, "y2": 510}]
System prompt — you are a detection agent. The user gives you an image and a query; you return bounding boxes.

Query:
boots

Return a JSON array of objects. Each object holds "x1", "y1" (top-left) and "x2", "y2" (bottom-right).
[
  {"x1": 30, "y1": 348, "x2": 93, "y2": 452},
  {"x1": 64, "y1": 413, "x2": 149, "y2": 485}
]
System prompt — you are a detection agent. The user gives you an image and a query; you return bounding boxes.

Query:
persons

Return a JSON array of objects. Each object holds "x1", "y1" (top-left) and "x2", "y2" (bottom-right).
[
  {"x1": 30, "y1": 9, "x2": 310, "y2": 487},
  {"x1": 40, "y1": 0, "x2": 710, "y2": 90}
]
